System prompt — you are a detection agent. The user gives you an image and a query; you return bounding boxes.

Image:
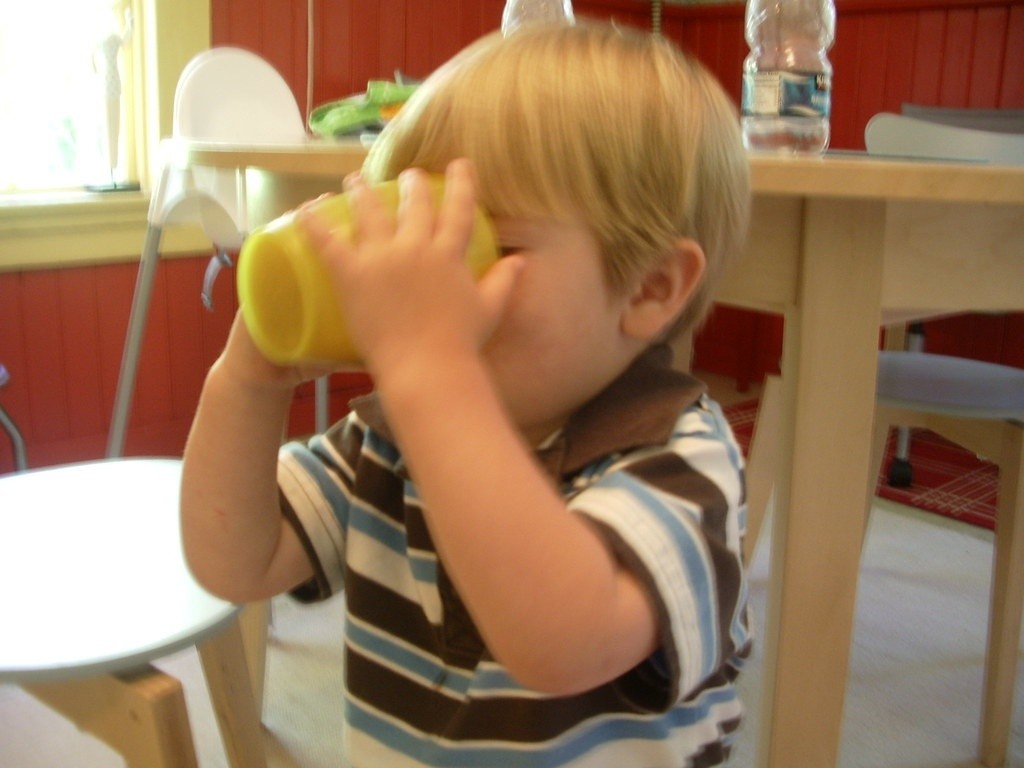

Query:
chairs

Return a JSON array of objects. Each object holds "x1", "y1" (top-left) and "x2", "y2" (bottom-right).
[
  {"x1": 740, "y1": 110, "x2": 1024, "y2": 767},
  {"x1": 1, "y1": 48, "x2": 308, "y2": 767}
]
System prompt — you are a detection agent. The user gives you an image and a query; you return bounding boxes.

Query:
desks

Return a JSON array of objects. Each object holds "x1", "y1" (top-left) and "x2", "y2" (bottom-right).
[{"x1": 189, "y1": 143, "x2": 1023, "y2": 767}]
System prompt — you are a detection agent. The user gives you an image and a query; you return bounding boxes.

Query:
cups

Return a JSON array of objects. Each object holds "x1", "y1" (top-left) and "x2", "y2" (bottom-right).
[{"x1": 235, "y1": 178, "x2": 496, "y2": 372}]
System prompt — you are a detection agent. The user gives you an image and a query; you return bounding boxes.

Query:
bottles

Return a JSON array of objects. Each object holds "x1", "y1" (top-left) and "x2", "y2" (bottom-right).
[
  {"x1": 503, "y1": 0, "x2": 572, "y2": 33},
  {"x1": 742, "y1": 0, "x2": 836, "y2": 156}
]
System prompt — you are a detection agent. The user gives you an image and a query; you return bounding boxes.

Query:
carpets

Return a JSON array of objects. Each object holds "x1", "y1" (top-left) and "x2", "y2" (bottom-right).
[{"x1": 724, "y1": 398, "x2": 999, "y2": 531}]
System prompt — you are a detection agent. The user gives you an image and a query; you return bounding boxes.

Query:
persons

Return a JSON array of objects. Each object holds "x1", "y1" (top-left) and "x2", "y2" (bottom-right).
[{"x1": 177, "y1": 19, "x2": 758, "y2": 768}]
